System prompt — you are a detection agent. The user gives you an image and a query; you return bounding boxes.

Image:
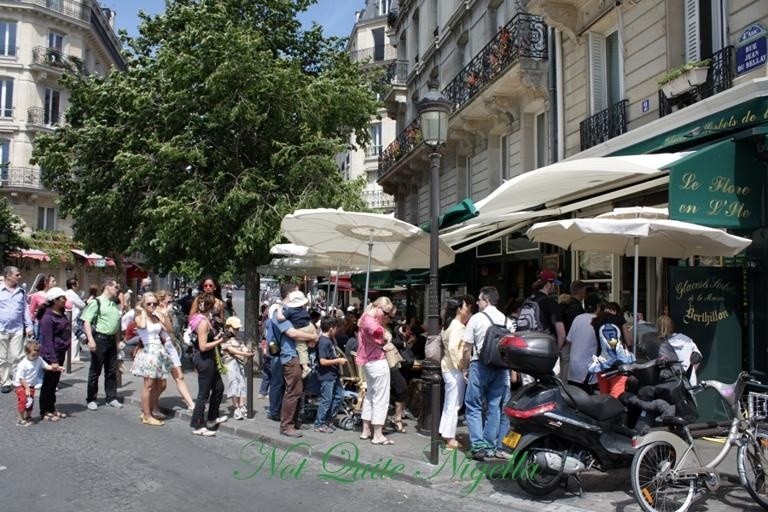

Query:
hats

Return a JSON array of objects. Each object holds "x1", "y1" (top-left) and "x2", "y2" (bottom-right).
[
  {"x1": 540, "y1": 270, "x2": 561, "y2": 285},
  {"x1": 141, "y1": 278, "x2": 152, "y2": 289},
  {"x1": 225, "y1": 316, "x2": 242, "y2": 329},
  {"x1": 286, "y1": 291, "x2": 309, "y2": 308},
  {"x1": 585, "y1": 294, "x2": 600, "y2": 304},
  {"x1": 46, "y1": 287, "x2": 65, "y2": 302}
]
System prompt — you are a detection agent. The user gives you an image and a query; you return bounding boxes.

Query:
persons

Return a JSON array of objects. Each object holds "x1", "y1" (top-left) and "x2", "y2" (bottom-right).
[{"x1": 0, "y1": 265, "x2": 704, "y2": 463}]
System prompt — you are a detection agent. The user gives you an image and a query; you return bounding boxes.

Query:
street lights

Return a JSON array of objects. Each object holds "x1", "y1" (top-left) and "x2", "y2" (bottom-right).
[{"x1": 414, "y1": 65, "x2": 453, "y2": 437}]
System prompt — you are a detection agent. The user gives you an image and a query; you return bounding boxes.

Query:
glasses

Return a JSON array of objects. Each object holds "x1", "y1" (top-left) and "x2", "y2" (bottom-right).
[
  {"x1": 204, "y1": 284, "x2": 214, "y2": 287},
  {"x1": 380, "y1": 307, "x2": 395, "y2": 320},
  {"x1": 146, "y1": 302, "x2": 158, "y2": 307}
]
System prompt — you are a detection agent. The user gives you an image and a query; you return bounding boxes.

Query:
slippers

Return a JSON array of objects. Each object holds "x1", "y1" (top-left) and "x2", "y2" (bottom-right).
[
  {"x1": 192, "y1": 426, "x2": 216, "y2": 437},
  {"x1": 389, "y1": 419, "x2": 409, "y2": 433},
  {"x1": 206, "y1": 415, "x2": 229, "y2": 429},
  {"x1": 359, "y1": 431, "x2": 395, "y2": 446}
]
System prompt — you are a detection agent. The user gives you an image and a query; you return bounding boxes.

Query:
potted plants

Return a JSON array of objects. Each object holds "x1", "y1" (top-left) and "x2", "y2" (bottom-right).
[{"x1": 656, "y1": 57, "x2": 710, "y2": 101}]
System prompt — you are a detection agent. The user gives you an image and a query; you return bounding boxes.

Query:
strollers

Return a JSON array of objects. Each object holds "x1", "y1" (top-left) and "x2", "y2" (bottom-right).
[{"x1": 332, "y1": 345, "x2": 408, "y2": 436}]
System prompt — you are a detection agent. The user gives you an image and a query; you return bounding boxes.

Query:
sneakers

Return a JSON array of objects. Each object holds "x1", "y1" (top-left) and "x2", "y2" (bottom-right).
[
  {"x1": 469, "y1": 447, "x2": 513, "y2": 462},
  {"x1": 188, "y1": 402, "x2": 208, "y2": 412},
  {"x1": 314, "y1": 421, "x2": 337, "y2": 434},
  {"x1": 257, "y1": 394, "x2": 265, "y2": 399},
  {"x1": 233, "y1": 406, "x2": 248, "y2": 420},
  {"x1": 87, "y1": 401, "x2": 98, "y2": 411},
  {"x1": 105, "y1": 399, "x2": 123, "y2": 409},
  {"x1": 16, "y1": 415, "x2": 35, "y2": 426}
]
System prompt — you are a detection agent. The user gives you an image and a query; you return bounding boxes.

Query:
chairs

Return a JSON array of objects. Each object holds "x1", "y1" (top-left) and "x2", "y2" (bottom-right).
[
  {"x1": 332, "y1": 345, "x2": 356, "y2": 389},
  {"x1": 349, "y1": 350, "x2": 369, "y2": 411}
]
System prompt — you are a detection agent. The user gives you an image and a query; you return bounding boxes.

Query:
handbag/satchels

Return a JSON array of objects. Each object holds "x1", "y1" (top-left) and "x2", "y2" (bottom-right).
[
  {"x1": 266, "y1": 319, "x2": 285, "y2": 358},
  {"x1": 123, "y1": 309, "x2": 142, "y2": 345},
  {"x1": 424, "y1": 334, "x2": 444, "y2": 365},
  {"x1": 188, "y1": 333, "x2": 198, "y2": 345},
  {"x1": 382, "y1": 339, "x2": 404, "y2": 369},
  {"x1": 72, "y1": 298, "x2": 101, "y2": 345},
  {"x1": 621, "y1": 310, "x2": 634, "y2": 347}
]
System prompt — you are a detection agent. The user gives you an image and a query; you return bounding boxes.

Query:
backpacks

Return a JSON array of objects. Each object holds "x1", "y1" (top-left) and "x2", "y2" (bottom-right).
[
  {"x1": 516, "y1": 295, "x2": 550, "y2": 335},
  {"x1": 473, "y1": 311, "x2": 510, "y2": 370}
]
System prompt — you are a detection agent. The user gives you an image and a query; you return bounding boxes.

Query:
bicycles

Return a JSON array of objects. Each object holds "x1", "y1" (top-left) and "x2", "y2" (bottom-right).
[
  {"x1": 629, "y1": 371, "x2": 767, "y2": 511},
  {"x1": 169, "y1": 310, "x2": 191, "y2": 369}
]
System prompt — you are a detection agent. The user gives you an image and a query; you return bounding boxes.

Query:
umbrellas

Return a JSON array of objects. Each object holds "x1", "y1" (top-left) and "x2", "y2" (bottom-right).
[
  {"x1": 269, "y1": 207, "x2": 456, "y2": 316},
  {"x1": 521, "y1": 206, "x2": 753, "y2": 367}
]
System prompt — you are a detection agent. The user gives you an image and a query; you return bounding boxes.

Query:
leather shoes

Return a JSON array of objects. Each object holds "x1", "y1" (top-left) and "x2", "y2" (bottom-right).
[
  {"x1": 279, "y1": 425, "x2": 303, "y2": 439},
  {"x1": 294, "y1": 423, "x2": 312, "y2": 430},
  {"x1": 139, "y1": 408, "x2": 167, "y2": 426},
  {"x1": 266, "y1": 412, "x2": 281, "y2": 421},
  {"x1": 2, "y1": 386, "x2": 11, "y2": 393}
]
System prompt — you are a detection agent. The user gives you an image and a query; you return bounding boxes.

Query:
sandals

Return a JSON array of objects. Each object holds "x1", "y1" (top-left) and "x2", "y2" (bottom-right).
[
  {"x1": 40, "y1": 410, "x2": 67, "y2": 422},
  {"x1": 436, "y1": 432, "x2": 463, "y2": 449}
]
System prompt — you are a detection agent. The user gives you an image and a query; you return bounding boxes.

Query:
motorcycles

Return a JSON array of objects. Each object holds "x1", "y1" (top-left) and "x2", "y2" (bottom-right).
[{"x1": 495, "y1": 326, "x2": 701, "y2": 500}]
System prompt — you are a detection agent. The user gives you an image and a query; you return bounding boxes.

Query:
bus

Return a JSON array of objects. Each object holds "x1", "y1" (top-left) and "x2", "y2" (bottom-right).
[{"x1": 221, "y1": 275, "x2": 280, "y2": 292}]
